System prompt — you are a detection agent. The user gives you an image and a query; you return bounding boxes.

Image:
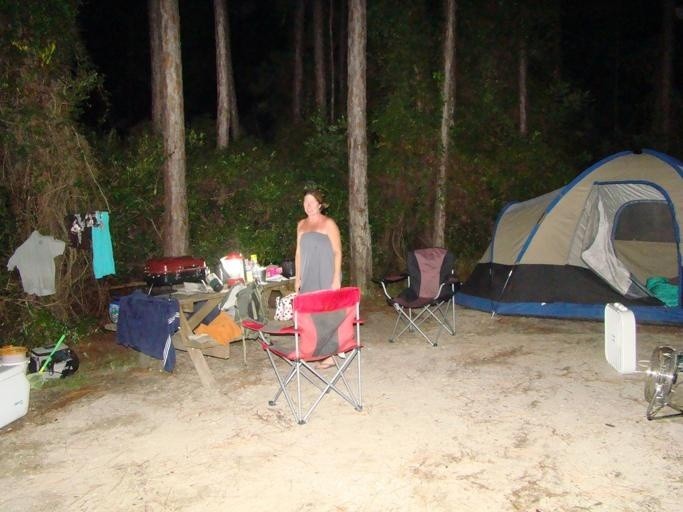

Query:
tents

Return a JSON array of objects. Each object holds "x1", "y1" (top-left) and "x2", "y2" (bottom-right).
[{"x1": 442, "y1": 136, "x2": 682, "y2": 330}]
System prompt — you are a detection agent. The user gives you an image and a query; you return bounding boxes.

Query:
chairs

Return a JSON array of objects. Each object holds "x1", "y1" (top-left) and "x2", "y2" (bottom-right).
[
  {"x1": 257, "y1": 286, "x2": 365, "y2": 424},
  {"x1": 371, "y1": 248, "x2": 464, "y2": 348}
]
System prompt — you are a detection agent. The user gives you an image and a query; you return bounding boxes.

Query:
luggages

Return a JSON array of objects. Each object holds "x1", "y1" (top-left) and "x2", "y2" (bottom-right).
[{"x1": 143, "y1": 256, "x2": 205, "y2": 286}]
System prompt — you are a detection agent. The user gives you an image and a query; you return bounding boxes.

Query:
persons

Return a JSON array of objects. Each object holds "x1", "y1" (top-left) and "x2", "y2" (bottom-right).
[{"x1": 291, "y1": 190, "x2": 345, "y2": 374}]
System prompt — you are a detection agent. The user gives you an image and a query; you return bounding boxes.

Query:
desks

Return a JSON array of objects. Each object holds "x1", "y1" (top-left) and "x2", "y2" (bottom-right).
[{"x1": 121, "y1": 276, "x2": 294, "y2": 387}]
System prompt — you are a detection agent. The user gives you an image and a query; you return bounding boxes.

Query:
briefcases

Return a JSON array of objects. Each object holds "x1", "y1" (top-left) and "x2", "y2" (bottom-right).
[{"x1": 604, "y1": 303, "x2": 637, "y2": 373}]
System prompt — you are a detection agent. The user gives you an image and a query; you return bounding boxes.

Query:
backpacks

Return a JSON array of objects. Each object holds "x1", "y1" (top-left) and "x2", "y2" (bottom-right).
[{"x1": 30, "y1": 344, "x2": 79, "y2": 379}]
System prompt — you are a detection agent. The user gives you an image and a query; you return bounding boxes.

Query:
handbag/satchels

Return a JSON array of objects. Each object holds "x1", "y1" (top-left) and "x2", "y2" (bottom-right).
[{"x1": 275, "y1": 293, "x2": 296, "y2": 322}]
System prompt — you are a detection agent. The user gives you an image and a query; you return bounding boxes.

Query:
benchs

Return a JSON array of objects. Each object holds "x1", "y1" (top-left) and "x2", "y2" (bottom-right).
[{"x1": 183, "y1": 315, "x2": 274, "y2": 360}]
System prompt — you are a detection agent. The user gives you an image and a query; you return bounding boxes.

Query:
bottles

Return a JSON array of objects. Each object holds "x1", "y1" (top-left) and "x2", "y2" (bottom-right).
[
  {"x1": 244, "y1": 264, "x2": 253, "y2": 284},
  {"x1": 205, "y1": 272, "x2": 223, "y2": 292},
  {"x1": 252, "y1": 260, "x2": 261, "y2": 284}
]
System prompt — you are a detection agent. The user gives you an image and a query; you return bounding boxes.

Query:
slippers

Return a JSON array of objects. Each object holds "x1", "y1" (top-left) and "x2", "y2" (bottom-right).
[{"x1": 314, "y1": 362, "x2": 335, "y2": 370}]
